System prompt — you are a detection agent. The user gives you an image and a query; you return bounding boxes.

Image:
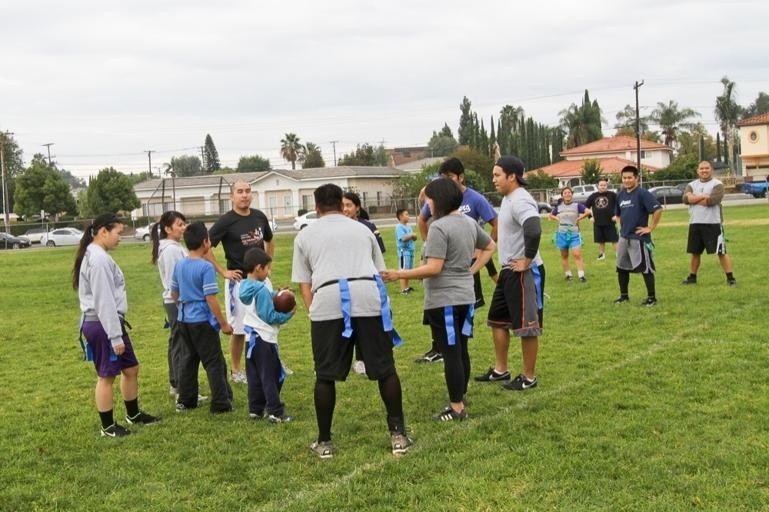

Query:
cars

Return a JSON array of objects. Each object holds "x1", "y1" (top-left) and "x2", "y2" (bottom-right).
[
  {"x1": 293, "y1": 210, "x2": 320, "y2": 229},
  {"x1": 268, "y1": 221, "x2": 278, "y2": 232},
  {"x1": 647, "y1": 186, "x2": 684, "y2": 204},
  {"x1": 536, "y1": 202, "x2": 551, "y2": 213},
  {"x1": 0, "y1": 232, "x2": 31, "y2": 249},
  {"x1": 40, "y1": 227, "x2": 85, "y2": 247},
  {"x1": 735, "y1": 184, "x2": 751, "y2": 194},
  {"x1": 134, "y1": 222, "x2": 161, "y2": 241},
  {"x1": 17, "y1": 229, "x2": 49, "y2": 242}
]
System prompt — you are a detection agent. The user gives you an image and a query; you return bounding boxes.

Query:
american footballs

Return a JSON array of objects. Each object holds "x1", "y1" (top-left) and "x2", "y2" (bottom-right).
[{"x1": 274, "y1": 289, "x2": 295, "y2": 313}]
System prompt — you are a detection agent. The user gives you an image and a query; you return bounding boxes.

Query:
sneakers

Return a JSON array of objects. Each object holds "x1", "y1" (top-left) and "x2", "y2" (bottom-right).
[
  {"x1": 596, "y1": 252, "x2": 605, "y2": 260},
  {"x1": 430, "y1": 406, "x2": 469, "y2": 421},
  {"x1": 614, "y1": 296, "x2": 629, "y2": 304},
  {"x1": 248, "y1": 401, "x2": 295, "y2": 423},
  {"x1": 414, "y1": 349, "x2": 444, "y2": 364},
  {"x1": 353, "y1": 360, "x2": 366, "y2": 375},
  {"x1": 499, "y1": 373, "x2": 538, "y2": 391},
  {"x1": 100, "y1": 421, "x2": 137, "y2": 438},
  {"x1": 170, "y1": 384, "x2": 234, "y2": 416},
  {"x1": 726, "y1": 278, "x2": 736, "y2": 285},
  {"x1": 391, "y1": 432, "x2": 417, "y2": 455},
  {"x1": 125, "y1": 409, "x2": 163, "y2": 426},
  {"x1": 564, "y1": 275, "x2": 587, "y2": 283},
  {"x1": 230, "y1": 368, "x2": 249, "y2": 384},
  {"x1": 681, "y1": 276, "x2": 696, "y2": 285},
  {"x1": 309, "y1": 440, "x2": 333, "y2": 459},
  {"x1": 473, "y1": 366, "x2": 511, "y2": 382},
  {"x1": 400, "y1": 287, "x2": 414, "y2": 295},
  {"x1": 641, "y1": 297, "x2": 657, "y2": 307}
]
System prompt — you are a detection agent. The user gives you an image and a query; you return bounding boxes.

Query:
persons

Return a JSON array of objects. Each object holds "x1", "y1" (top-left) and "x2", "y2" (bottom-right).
[
  {"x1": 171, "y1": 220, "x2": 238, "y2": 415},
  {"x1": 289, "y1": 183, "x2": 416, "y2": 458},
  {"x1": 683, "y1": 160, "x2": 736, "y2": 285},
  {"x1": 613, "y1": 166, "x2": 663, "y2": 307},
  {"x1": 151, "y1": 211, "x2": 209, "y2": 402},
  {"x1": 342, "y1": 159, "x2": 498, "y2": 422},
  {"x1": 549, "y1": 187, "x2": 591, "y2": 282},
  {"x1": 232, "y1": 247, "x2": 293, "y2": 424},
  {"x1": 474, "y1": 154, "x2": 546, "y2": 391},
  {"x1": 203, "y1": 179, "x2": 294, "y2": 385},
  {"x1": 584, "y1": 179, "x2": 619, "y2": 260},
  {"x1": 71, "y1": 213, "x2": 161, "y2": 438}
]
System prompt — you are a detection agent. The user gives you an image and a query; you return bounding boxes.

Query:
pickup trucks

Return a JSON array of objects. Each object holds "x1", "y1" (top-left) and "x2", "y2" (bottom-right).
[
  {"x1": 744, "y1": 181, "x2": 769, "y2": 198},
  {"x1": 549, "y1": 184, "x2": 618, "y2": 205}
]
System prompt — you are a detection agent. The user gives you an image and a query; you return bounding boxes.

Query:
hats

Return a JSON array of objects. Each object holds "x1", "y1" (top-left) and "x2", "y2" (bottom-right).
[{"x1": 495, "y1": 156, "x2": 529, "y2": 186}]
